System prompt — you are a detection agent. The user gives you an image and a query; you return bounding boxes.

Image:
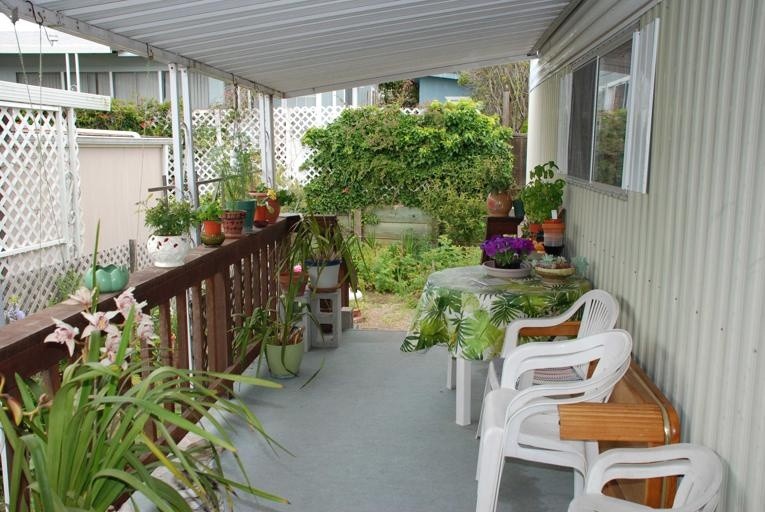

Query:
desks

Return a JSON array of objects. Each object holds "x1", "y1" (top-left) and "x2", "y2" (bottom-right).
[{"x1": 400, "y1": 268, "x2": 596, "y2": 426}]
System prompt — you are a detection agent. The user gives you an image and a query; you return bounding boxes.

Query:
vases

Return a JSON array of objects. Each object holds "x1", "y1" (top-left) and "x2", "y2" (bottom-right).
[
  {"x1": 279, "y1": 272, "x2": 309, "y2": 298},
  {"x1": 539, "y1": 215, "x2": 567, "y2": 257},
  {"x1": 84, "y1": 264, "x2": 129, "y2": 292},
  {"x1": 493, "y1": 257, "x2": 522, "y2": 270}
]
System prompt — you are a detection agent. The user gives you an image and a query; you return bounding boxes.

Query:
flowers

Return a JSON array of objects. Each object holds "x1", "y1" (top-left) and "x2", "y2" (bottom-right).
[
  {"x1": 482, "y1": 236, "x2": 535, "y2": 261},
  {"x1": 0, "y1": 217, "x2": 295, "y2": 511},
  {"x1": 291, "y1": 259, "x2": 302, "y2": 273}
]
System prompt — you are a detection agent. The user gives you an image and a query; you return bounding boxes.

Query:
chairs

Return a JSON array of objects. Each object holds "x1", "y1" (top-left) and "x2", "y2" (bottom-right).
[
  {"x1": 476, "y1": 328, "x2": 634, "y2": 512},
  {"x1": 568, "y1": 441, "x2": 724, "y2": 512},
  {"x1": 475, "y1": 289, "x2": 622, "y2": 437}
]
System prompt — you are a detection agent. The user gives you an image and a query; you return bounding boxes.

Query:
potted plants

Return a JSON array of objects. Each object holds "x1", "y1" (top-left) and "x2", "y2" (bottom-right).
[
  {"x1": 227, "y1": 256, "x2": 328, "y2": 391},
  {"x1": 483, "y1": 153, "x2": 514, "y2": 217},
  {"x1": 197, "y1": 141, "x2": 285, "y2": 248},
  {"x1": 521, "y1": 161, "x2": 565, "y2": 238},
  {"x1": 272, "y1": 190, "x2": 372, "y2": 316},
  {"x1": 536, "y1": 252, "x2": 575, "y2": 284},
  {"x1": 136, "y1": 195, "x2": 197, "y2": 268}
]
